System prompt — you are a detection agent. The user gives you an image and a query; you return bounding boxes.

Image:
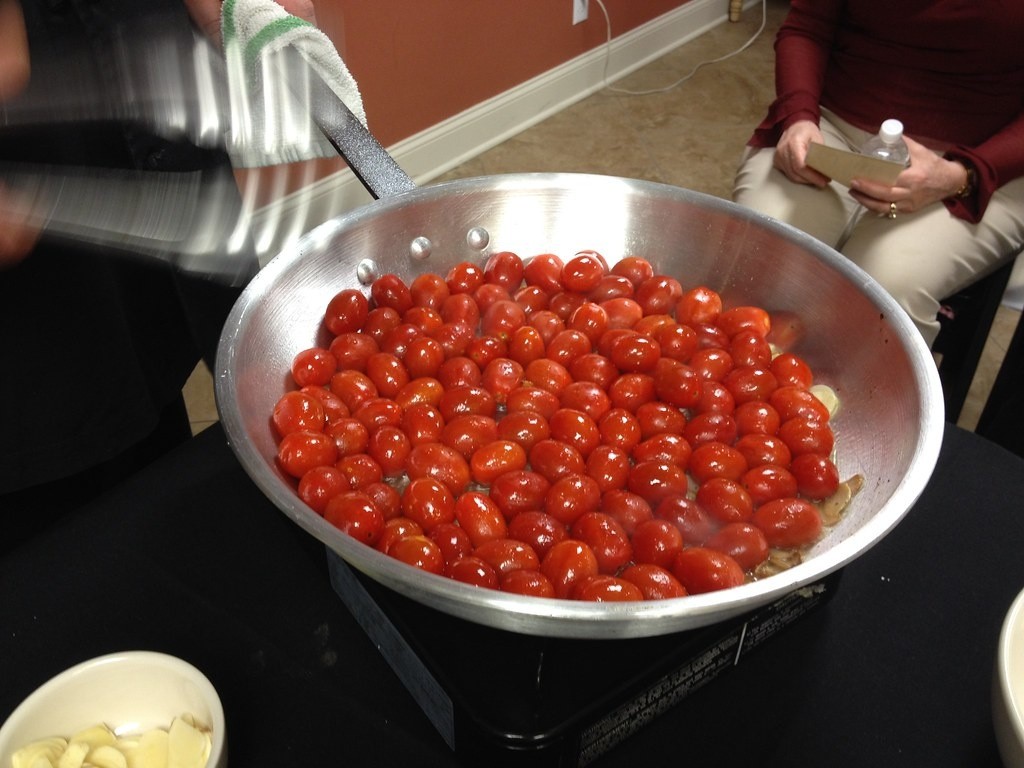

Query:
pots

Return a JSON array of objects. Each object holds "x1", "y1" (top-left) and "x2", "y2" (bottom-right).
[{"x1": 214, "y1": 43, "x2": 945, "y2": 639}]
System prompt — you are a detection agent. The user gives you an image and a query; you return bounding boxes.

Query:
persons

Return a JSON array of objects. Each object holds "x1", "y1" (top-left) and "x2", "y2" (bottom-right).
[
  {"x1": 0, "y1": 0, "x2": 316, "y2": 559},
  {"x1": 733, "y1": 0, "x2": 1024, "y2": 351}
]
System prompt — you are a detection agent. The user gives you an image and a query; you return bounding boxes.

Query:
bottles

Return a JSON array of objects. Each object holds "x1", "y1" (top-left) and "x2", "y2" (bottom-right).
[{"x1": 861, "y1": 119, "x2": 911, "y2": 165}]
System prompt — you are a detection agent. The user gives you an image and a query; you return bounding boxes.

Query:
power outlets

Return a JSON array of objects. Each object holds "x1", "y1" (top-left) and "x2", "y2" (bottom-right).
[{"x1": 573, "y1": 0, "x2": 589, "y2": 25}]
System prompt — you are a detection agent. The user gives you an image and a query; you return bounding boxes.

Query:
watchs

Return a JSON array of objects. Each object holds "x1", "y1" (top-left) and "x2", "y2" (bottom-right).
[{"x1": 958, "y1": 159, "x2": 976, "y2": 198}]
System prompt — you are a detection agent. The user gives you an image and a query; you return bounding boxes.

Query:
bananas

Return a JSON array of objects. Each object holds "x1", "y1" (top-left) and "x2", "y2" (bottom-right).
[{"x1": 11, "y1": 718, "x2": 214, "y2": 768}]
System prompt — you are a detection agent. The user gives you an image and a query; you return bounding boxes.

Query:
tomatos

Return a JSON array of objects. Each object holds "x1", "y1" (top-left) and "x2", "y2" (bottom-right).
[{"x1": 273, "y1": 252, "x2": 838, "y2": 603}]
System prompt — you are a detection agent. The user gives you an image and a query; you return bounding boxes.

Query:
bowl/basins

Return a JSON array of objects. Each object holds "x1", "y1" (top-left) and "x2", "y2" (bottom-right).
[{"x1": 0, "y1": 651, "x2": 225, "y2": 768}]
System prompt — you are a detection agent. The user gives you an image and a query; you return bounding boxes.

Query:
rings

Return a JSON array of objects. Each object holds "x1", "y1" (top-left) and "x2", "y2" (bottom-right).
[{"x1": 888, "y1": 201, "x2": 897, "y2": 219}]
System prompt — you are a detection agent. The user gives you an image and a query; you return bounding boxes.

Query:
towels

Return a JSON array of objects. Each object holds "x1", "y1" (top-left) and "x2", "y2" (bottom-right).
[{"x1": 218, "y1": 0, "x2": 370, "y2": 169}]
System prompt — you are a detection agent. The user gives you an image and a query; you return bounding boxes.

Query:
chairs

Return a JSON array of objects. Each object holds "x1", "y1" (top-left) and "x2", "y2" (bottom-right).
[{"x1": 931, "y1": 249, "x2": 1024, "y2": 431}]
